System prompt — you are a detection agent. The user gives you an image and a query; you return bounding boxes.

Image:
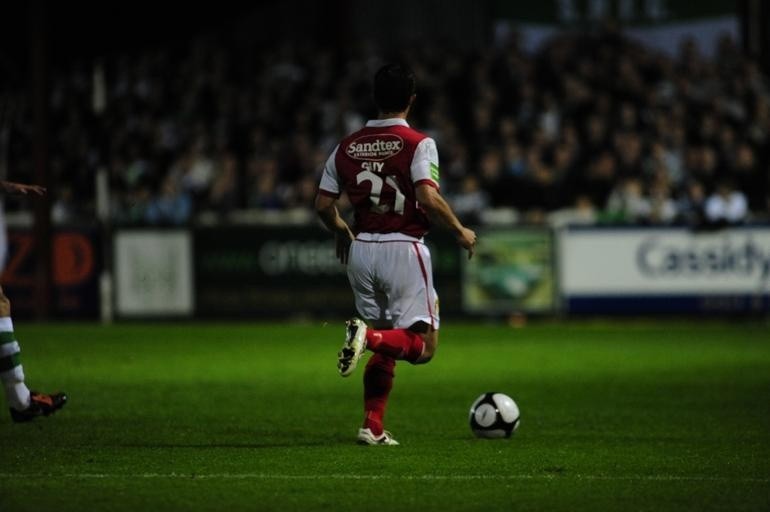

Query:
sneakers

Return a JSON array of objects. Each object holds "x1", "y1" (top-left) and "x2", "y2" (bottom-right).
[
  {"x1": 7, "y1": 389, "x2": 69, "y2": 423},
  {"x1": 355, "y1": 424, "x2": 402, "y2": 447},
  {"x1": 336, "y1": 315, "x2": 369, "y2": 379}
]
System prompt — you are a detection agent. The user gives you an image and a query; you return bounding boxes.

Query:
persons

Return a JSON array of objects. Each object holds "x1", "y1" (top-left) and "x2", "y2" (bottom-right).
[
  {"x1": 315, "y1": 65, "x2": 476, "y2": 446},
  {"x1": 1, "y1": 1, "x2": 769, "y2": 226},
  {"x1": 0, "y1": 179, "x2": 67, "y2": 423}
]
